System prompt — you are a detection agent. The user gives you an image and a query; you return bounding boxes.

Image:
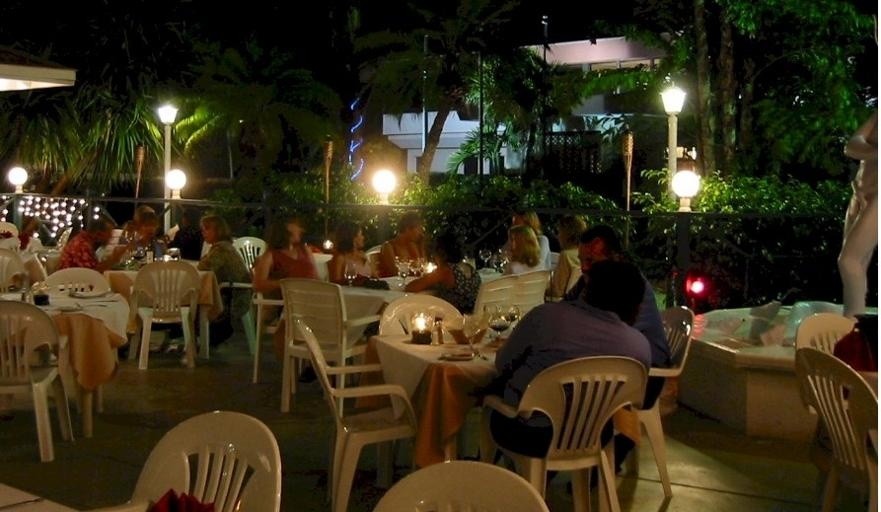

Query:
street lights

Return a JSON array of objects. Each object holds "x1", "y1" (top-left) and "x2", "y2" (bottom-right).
[
  {"x1": 667, "y1": 153, "x2": 701, "y2": 307},
  {"x1": 371, "y1": 168, "x2": 400, "y2": 241},
  {"x1": 162, "y1": 166, "x2": 189, "y2": 228},
  {"x1": 155, "y1": 102, "x2": 180, "y2": 240},
  {"x1": 656, "y1": 82, "x2": 686, "y2": 208},
  {"x1": 7, "y1": 166, "x2": 30, "y2": 231}
]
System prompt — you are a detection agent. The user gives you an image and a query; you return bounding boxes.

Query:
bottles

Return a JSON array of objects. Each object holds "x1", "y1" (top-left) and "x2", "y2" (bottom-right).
[
  {"x1": 434, "y1": 315, "x2": 444, "y2": 345},
  {"x1": 145, "y1": 243, "x2": 155, "y2": 265},
  {"x1": 21, "y1": 287, "x2": 34, "y2": 306}
]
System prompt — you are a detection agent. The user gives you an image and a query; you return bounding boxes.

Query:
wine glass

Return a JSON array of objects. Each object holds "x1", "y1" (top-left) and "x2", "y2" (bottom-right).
[
  {"x1": 396, "y1": 255, "x2": 429, "y2": 288},
  {"x1": 125, "y1": 230, "x2": 136, "y2": 263},
  {"x1": 463, "y1": 303, "x2": 527, "y2": 355},
  {"x1": 345, "y1": 263, "x2": 359, "y2": 287},
  {"x1": 463, "y1": 244, "x2": 512, "y2": 276},
  {"x1": 169, "y1": 247, "x2": 181, "y2": 261}
]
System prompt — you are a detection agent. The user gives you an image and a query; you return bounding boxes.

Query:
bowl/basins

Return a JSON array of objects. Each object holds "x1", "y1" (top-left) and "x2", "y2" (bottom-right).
[{"x1": 447, "y1": 327, "x2": 487, "y2": 344}]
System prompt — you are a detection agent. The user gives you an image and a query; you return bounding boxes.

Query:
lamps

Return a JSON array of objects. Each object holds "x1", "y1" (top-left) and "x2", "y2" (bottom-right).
[
  {"x1": 8, "y1": 167, "x2": 28, "y2": 194},
  {"x1": 372, "y1": 169, "x2": 396, "y2": 205},
  {"x1": 164, "y1": 169, "x2": 187, "y2": 200},
  {"x1": 670, "y1": 170, "x2": 700, "y2": 212}
]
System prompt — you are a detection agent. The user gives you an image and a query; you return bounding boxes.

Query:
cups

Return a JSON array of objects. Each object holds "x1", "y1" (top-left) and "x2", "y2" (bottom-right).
[
  {"x1": 323, "y1": 240, "x2": 334, "y2": 254},
  {"x1": 35, "y1": 292, "x2": 49, "y2": 306},
  {"x1": 409, "y1": 311, "x2": 434, "y2": 345}
]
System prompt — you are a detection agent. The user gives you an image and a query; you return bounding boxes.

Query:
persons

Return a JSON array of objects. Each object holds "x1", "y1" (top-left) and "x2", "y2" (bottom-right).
[
  {"x1": 379, "y1": 212, "x2": 430, "y2": 278},
  {"x1": 329, "y1": 220, "x2": 379, "y2": 285},
  {"x1": 0, "y1": 198, "x2": 42, "y2": 250},
  {"x1": 406, "y1": 229, "x2": 483, "y2": 315},
  {"x1": 250, "y1": 213, "x2": 336, "y2": 392},
  {"x1": 838, "y1": 109, "x2": 878, "y2": 318},
  {"x1": 58, "y1": 204, "x2": 253, "y2": 357},
  {"x1": 466, "y1": 208, "x2": 672, "y2": 493}
]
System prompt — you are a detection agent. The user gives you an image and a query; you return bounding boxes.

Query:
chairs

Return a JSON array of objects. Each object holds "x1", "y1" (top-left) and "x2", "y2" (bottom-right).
[
  {"x1": 793, "y1": 342, "x2": 879, "y2": 511},
  {"x1": 795, "y1": 313, "x2": 862, "y2": 433}
]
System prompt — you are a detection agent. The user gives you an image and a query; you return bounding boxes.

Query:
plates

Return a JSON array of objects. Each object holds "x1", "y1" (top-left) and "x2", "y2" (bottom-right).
[{"x1": 441, "y1": 351, "x2": 474, "y2": 360}]
